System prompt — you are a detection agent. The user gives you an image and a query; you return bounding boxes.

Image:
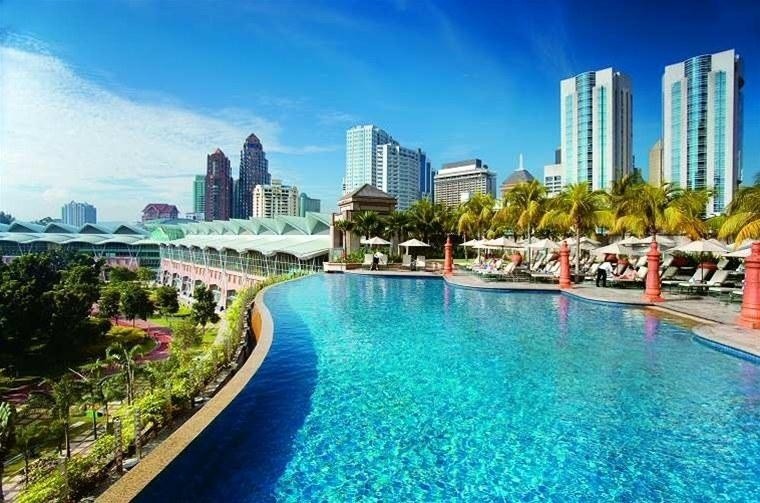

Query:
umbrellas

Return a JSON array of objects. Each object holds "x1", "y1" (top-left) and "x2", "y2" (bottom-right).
[
  {"x1": 457, "y1": 232, "x2": 752, "y2": 283},
  {"x1": 362, "y1": 235, "x2": 392, "y2": 244},
  {"x1": 397, "y1": 238, "x2": 432, "y2": 247}
]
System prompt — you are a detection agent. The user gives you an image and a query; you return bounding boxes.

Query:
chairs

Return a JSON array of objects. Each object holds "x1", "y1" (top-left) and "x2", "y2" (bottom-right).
[
  {"x1": 458, "y1": 251, "x2": 748, "y2": 307},
  {"x1": 363, "y1": 252, "x2": 388, "y2": 271},
  {"x1": 400, "y1": 253, "x2": 426, "y2": 270}
]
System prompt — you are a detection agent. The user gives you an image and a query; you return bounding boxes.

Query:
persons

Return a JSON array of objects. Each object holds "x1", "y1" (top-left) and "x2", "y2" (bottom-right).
[{"x1": 596, "y1": 261, "x2": 620, "y2": 287}]
